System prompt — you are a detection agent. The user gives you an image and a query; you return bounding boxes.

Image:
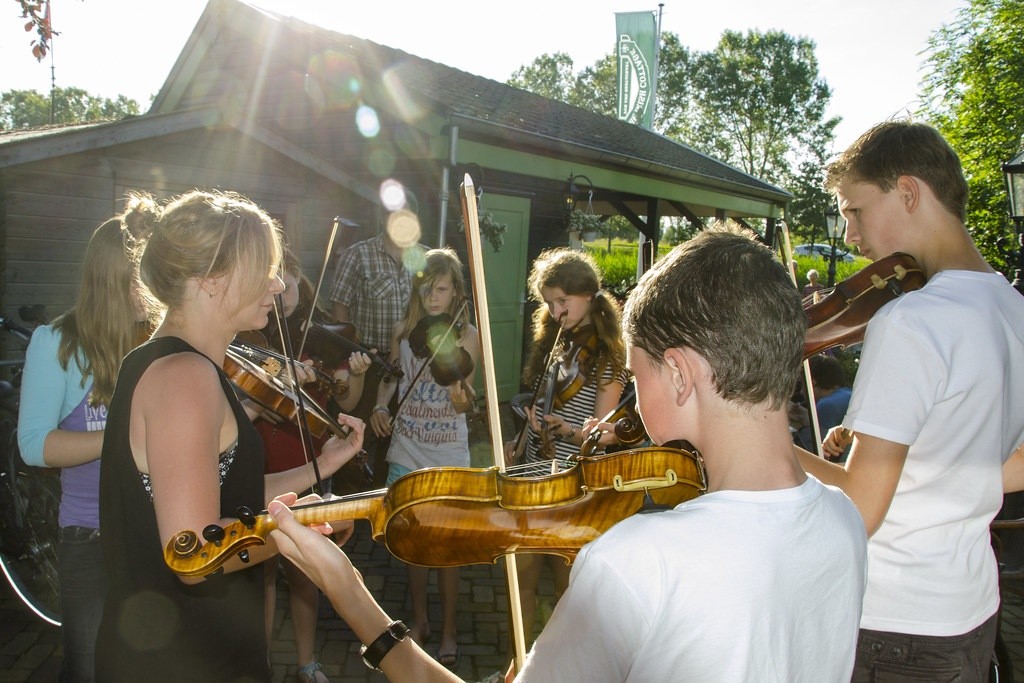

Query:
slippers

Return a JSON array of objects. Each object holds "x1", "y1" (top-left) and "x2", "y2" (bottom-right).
[{"x1": 431, "y1": 643, "x2": 461, "y2": 671}]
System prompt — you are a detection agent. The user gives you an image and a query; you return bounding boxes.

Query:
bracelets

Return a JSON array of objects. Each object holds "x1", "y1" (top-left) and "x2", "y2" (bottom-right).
[
  {"x1": 358, "y1": 620, "x2": 413, "y2": 675},
  {"x1": 562, "y1": 425, "x2": 575, "y2": 439},
  {"x1": 374, "y1": 403, "x2": 389, "y2": 415}
]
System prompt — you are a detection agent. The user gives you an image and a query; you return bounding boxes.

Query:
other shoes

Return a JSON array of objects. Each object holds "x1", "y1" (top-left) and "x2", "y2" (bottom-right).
[{"x1": 298, "y1": 661, "x2": 326, "y2": 683}]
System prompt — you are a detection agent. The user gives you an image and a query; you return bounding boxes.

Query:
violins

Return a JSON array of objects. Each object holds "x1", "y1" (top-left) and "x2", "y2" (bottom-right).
[
  {"x1": 532, "y1": 323, "x2": 601, "y2": 458},
  {"x1": 165, "y1": 447, "x2": 703, "y2": 577},
  {"x1": 614, "y1": 252, "x2": 926, "y2": 446},
  {"x1": 408, "y1": 313, "x2": 479, "y2": 413},
  {"x1": 133, "y1": 322, "x2": 348, "y2": 402},
  {"x1": 224, "y1": 352, "x2": 370, "y2": 466},
  {"x1": 272, "y1": 307, "x2": 406, "y2": 379}
]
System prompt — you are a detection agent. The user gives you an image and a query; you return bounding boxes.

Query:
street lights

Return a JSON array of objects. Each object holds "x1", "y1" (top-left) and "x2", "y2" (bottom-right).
[
  {"x1": 998, "y1": 150, "x2": 1024, "y2": 290},
  {"x1": 823, "y1": 206, "x2": 846, "y2": 287}
]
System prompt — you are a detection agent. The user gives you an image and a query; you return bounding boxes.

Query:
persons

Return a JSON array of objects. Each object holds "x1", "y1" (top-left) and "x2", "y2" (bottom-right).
[
  {"x1": 262, "y1": 231, "x2": 869, "y2": 683},
  {"x1": 787, "y1": 121, "x2": 1024, "y2": 683},
  {"x1": 249, "y1": 184, "x2": 480, "y2": 683},
  {"x1": 18, "y1": 214, "x2": 163, "y2": 683},
  {"x1": 101, "y1": 187, "x2": 369, "y2": 682},
  {"x1": 503, "y1": 246, "x2": 632, "y2": 675},
  {"x1": 790, "y1": 349, "x2": 854, "y2": 435},
  {"x1": 577, "y1": 211, "x2": 760, "y2": 454}
]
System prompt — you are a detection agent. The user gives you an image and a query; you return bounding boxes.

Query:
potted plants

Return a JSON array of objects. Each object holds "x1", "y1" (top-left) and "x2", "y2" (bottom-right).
[
  {"x1": 459, "y1": 211, "x2": 508, "y2": 253},
  {"x1": 565, "y1": 209, "x2": 616, "y2": 241}
]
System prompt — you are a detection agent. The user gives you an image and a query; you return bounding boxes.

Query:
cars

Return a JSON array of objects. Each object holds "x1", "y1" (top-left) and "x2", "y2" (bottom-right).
[{"x1": 795, "y1": 244, "x2": 855, "y2": 263}]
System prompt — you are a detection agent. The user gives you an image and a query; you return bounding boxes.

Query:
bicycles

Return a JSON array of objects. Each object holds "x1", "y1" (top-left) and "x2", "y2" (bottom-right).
[{"x1": 0, "y1": 302, "x2": 107, "y2": 630}]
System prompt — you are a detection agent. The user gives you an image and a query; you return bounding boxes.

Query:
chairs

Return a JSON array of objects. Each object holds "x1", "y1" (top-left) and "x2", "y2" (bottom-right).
[{"x1": 989, "y1": 516, "x2": 1024, "y2": 606}]
